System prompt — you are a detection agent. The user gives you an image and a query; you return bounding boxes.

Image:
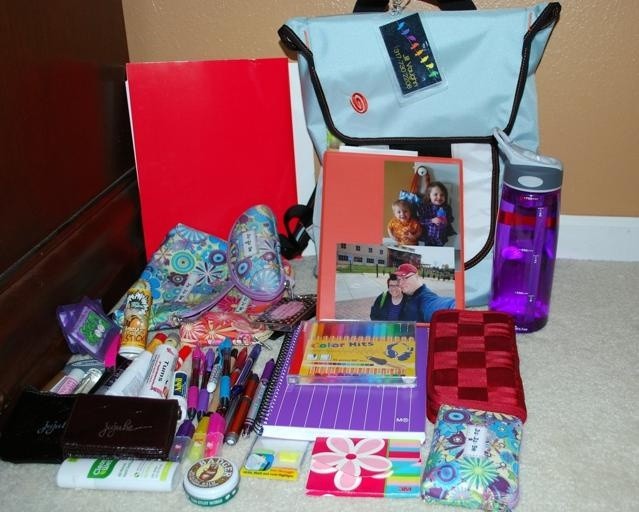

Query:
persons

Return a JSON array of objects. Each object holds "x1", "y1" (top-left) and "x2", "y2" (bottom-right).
[
  {"x1": 368, "y1": 275, "x2": 406, "y2": 321},
  {"x1": 387, "y1": 199, "x2": 421, "y2": 246},
  {"x1": 391, "y1": 263, "x2": 455, "y2": 323},
  {"x1": 416, "y1": 180, "x2": 455, "y2": 249}
]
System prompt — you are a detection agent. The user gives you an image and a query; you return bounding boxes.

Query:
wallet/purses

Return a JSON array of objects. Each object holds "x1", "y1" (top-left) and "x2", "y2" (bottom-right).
[
  {"x1": 419, "y1": 402, "x2": 524, "y2": 512},
  {"x1": 226, "y1": 202, "x2": 289, "y2": 302},
  {"x1": 425, "y1": 306, "x2": 529, "y2": 426}
]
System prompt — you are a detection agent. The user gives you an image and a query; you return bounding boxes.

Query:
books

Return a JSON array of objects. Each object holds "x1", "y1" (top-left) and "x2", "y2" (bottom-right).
[
  {"x1": 304, "y1": 437, "x2": 422, "y2": 499},
  {"x1": 254, "y1": 319, "x2": 428, "y2": 442}
]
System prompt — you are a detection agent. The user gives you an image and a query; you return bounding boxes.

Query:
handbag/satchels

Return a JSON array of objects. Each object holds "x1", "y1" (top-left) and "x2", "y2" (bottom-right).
[
  {"x1": 275, "y1": 1, "x2": 563, "y2": 311},
  {"x1": 112, "y1": 222, "x2": 236, "y2": 332}
]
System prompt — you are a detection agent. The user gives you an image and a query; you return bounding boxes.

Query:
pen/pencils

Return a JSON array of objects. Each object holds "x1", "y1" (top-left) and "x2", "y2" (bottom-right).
[{"x1": 186, "y1": 338, "x2": 275, "y2": 444}]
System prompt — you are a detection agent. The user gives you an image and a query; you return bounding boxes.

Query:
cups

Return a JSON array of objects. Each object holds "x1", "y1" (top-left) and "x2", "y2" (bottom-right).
[{"x1": 486, "y1": 125, "x2": 564, "y2": 334}]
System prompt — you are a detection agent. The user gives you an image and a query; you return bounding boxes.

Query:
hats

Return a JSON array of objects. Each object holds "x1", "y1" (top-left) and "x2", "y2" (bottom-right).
[{"x1": 391, "y1": 263, "x2": 417, "y2": 276}]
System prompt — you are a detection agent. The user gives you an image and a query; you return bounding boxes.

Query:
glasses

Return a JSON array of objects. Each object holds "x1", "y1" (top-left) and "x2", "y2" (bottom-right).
[{"x1": 397, "y1": 274, "x2": 416, "y2": 282}]
